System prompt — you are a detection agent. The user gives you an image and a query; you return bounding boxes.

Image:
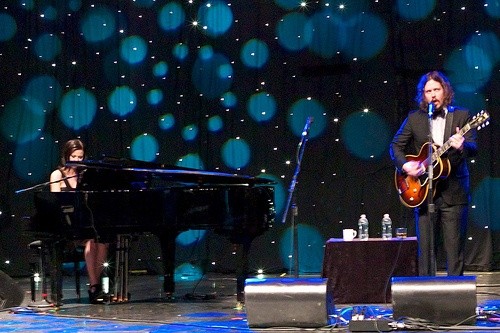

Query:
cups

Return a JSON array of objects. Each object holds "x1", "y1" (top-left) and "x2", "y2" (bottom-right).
[
  {"x1": 342, "y1": 228, "x2": 357, "y2": 241},
  {"x1": 396, "y1": 228, "x2": 407, "y2": 239}
]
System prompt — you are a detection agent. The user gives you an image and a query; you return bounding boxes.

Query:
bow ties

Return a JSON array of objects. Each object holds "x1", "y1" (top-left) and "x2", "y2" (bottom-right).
[{"x1": 431, "y1": 109, "x2": 446, "y2": 119}]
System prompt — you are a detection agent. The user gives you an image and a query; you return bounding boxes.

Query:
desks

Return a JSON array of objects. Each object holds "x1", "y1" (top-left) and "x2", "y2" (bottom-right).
[{"x1": 322, "y1": 236, "x2": 419, "y2": 303}]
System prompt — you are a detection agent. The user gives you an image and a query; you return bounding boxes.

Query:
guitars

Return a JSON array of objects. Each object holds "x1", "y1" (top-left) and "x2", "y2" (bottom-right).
[{"x1": 395, "y1": 110, "x2": 490, "y2": 208}]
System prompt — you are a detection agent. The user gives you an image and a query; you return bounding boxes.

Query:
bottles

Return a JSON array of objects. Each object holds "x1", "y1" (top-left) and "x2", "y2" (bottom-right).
[
  {"x1": 381, "y1": 214, "x2": 392, "y2": 239},
  {"x1": 358, "y1": 214, "x2": 369, "y2": 241}
]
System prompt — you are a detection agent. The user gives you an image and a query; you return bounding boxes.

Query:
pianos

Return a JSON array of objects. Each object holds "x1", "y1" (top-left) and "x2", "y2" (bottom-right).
[{"x1": 22, "y1": 162, "x2": 278, "y2": 310}]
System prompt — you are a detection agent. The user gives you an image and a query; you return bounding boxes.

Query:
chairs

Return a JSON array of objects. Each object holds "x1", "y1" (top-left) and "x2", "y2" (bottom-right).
[{"x1": 22, "y1": 233, "x2": 88, "y2": 311}]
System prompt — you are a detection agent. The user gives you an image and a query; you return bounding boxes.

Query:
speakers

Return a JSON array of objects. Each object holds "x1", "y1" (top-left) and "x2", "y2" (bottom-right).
[
  {"x1": 0, "y1": 270, "x2": 26, "y2": 310},
  {"x1": 245, "y1": 277, "x2": 339, "y2": 328},
  {"x1": 391, "y1": 275, "x2": 477, "y2": 325}
]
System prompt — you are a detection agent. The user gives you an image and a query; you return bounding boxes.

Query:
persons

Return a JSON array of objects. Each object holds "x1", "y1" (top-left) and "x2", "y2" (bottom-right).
[
  {"x1": 389, "y1": 71, "x2": 478, "y2": 278},
  {"x1": 49, "y1": 138, "x2": 110, "y2": 303}
]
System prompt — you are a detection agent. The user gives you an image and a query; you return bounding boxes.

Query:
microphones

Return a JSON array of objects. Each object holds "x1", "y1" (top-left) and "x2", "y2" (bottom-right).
[
  {"x1": 428, "y1": 102, "x2": 434, "y2": 117},
  {"x1": 302, "y1": 116, "x2": 314, "y2": 137}
]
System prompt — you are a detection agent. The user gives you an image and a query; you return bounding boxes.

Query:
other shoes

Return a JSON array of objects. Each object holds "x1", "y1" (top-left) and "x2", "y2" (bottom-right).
[{"x1": 88, "y1": 283, "x2": 110, "y2": 304}]
